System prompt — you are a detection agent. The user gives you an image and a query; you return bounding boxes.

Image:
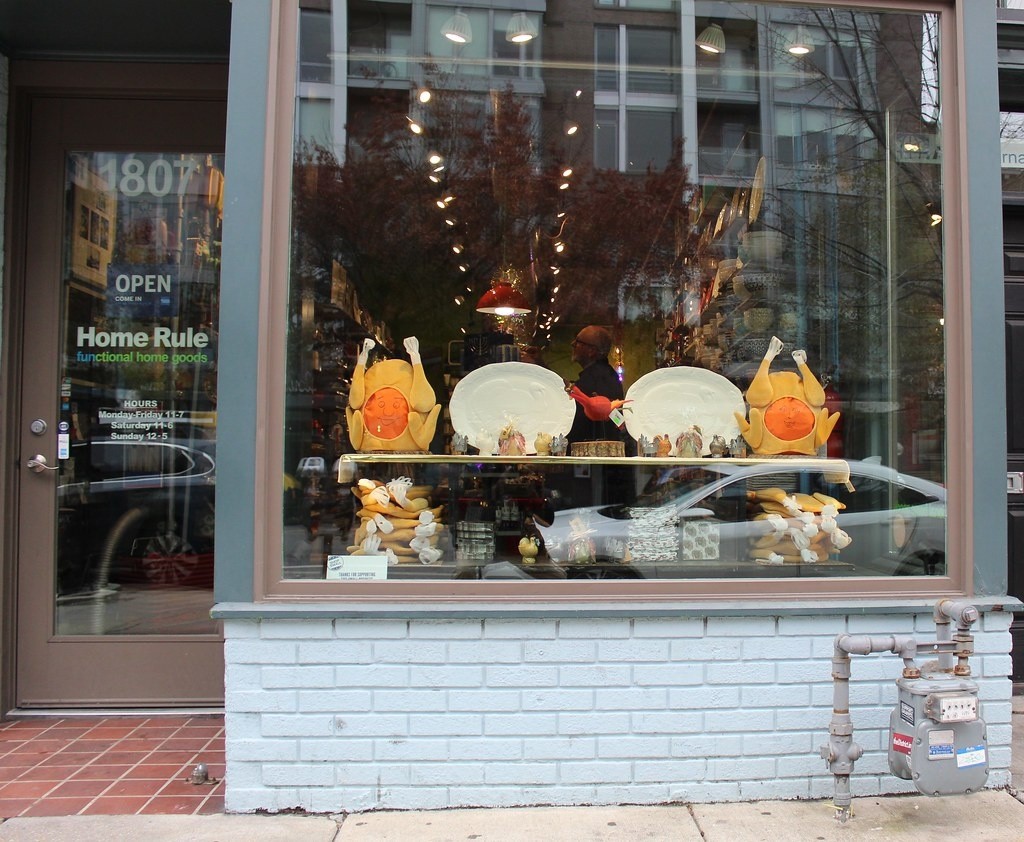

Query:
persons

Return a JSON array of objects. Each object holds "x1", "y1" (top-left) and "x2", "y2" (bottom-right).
[
  {"x1": 561, "y1": 326, "x2": 638, "y2": 510},
  {"x1": 482, "y1": 463, "x2": 556, "y2": 558}
]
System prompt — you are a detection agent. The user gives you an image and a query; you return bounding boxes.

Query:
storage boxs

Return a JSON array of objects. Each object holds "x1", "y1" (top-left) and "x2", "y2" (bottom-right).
[{"x1": 684, "y1": 521, "x2": 720, "y2": 559}]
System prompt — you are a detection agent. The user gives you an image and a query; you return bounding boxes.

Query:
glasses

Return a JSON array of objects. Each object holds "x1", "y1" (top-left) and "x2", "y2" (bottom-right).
[{"x1": 574, "y1": 334, "x2": 593, "y2": 349}]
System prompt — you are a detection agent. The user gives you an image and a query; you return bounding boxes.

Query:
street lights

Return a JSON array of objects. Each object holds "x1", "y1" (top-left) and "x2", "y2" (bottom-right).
[{"x1": 881, "y1": 78, "x2": 925, "y2": 550}]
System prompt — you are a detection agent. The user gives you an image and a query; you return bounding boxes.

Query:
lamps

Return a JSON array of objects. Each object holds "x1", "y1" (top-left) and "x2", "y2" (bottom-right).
[
  {"x1": 438, "y1": 6, "x2": 472, "y2": 43},
  {"x1": 693, "y1": 20, "x2": 725, "y2": 54},
  {"x1": 783, "y1": 24, "x2": 816, "y2": 55},
  {"x1": 475, "y1": 278, "x2": 533, "y2": 314},
  {"x1": 505, "y1": 11, "x2": 538, "y2": 42}
]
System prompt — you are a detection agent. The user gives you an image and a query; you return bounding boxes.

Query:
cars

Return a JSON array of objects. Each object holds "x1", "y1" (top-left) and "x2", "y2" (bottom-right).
[
  {"x1": 523, "y1": 459, "x2": 945, "y2": 576},
  {"x1": 56, "y1": 420, "x2": 215, "y2": 587}
]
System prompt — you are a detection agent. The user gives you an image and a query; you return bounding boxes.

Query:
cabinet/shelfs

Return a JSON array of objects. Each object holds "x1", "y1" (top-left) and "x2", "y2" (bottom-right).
[
  {"x1": 667, "y1": 128, "x2": 824, "y2": 382},
  {"x1": 318, "y1": 304, "x2": 398, "y2": 519}
]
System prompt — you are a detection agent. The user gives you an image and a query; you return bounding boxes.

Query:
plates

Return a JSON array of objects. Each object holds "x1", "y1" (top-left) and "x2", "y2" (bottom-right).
[
  {"x1": 621, "y1": 365, "x2": 746, "y2": 456},
  {"x1": 449, "y1": 362, "x2": 576, "y2": 456}
]
